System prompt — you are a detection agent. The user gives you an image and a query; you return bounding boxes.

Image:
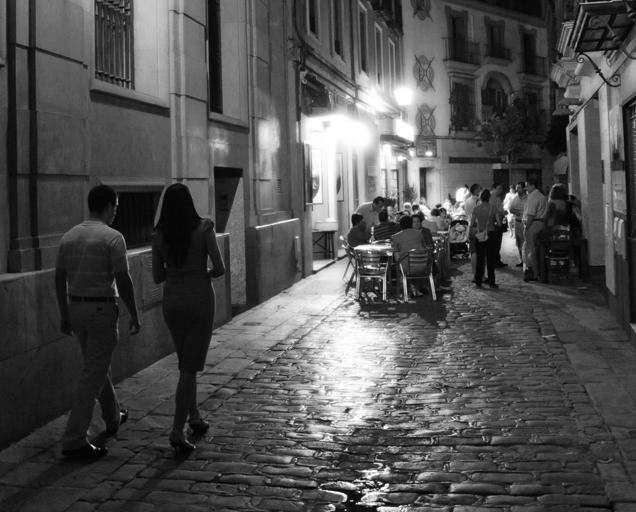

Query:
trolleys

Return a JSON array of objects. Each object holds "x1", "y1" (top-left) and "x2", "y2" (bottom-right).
[{"x1": 538, "y1": 229, "x2": 586, "y2": 283}]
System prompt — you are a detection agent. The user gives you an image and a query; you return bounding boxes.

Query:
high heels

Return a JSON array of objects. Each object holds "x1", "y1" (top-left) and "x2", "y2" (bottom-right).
[
  {"x1": 188, "y1": 420, "x2": 209, "y2": 436},
  {"x1": 169, "y1": 434, "x2": 196, "y2": 452}
]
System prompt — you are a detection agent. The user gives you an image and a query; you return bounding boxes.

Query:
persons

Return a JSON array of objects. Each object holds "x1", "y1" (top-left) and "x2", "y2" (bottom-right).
[
  {"x1": 150, "y1": 182, "x2": 226, "y2": 452},
  {"x1": 338, "y1": 173, "x2": 594, "y2": 305},
  {"x1": 56, "y1": 186, "x2": 142, "y2": 462}
]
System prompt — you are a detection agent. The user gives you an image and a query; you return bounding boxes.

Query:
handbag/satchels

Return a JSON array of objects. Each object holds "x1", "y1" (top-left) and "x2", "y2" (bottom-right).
[{"x1": 475, "y1": 231, "x2": 488, "y2": 242}]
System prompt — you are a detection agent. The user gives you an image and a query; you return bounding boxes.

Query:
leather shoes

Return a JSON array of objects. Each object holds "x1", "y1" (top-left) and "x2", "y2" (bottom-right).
[
  {"x1": 106, "y1": 409, "x2": 128, "y2": 434},
  {"x1": 472, "y1": 261, "x2": 538, "y2": 288},
  {"x1": 62, "y1": 444, "x2": 108, "y2": 457}
]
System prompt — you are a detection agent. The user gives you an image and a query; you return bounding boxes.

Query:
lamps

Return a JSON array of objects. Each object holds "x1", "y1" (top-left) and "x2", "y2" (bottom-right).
[{"x1": 575, "y1": 53, "x2": 621, "y2": 89}]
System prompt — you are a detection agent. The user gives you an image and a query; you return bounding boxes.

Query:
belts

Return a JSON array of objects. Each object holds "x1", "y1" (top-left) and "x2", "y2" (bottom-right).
[
  {"x1": 532, "y1": 218, "x2": 545, "y2": 221},
  {"x1": 67, "y1": 296, "x2": 117, "y2": 303},
  {"x1": 515, "y1": 218, "x2": 522, "y2": 222}
]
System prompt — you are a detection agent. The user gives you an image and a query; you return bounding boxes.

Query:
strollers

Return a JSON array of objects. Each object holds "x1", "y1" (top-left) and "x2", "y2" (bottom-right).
[{"x1": 449, "y1": 219, "x2": 469, "y2": 259}]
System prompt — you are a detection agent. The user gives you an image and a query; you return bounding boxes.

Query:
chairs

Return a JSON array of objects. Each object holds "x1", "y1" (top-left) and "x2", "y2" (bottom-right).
[{"x1": 339, "y1": 211, "x2": 470, "y2": 306}]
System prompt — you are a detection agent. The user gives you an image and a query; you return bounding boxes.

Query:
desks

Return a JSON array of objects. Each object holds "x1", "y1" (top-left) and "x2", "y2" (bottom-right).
[{"x1": 310, "y1": 229, "x2": 338, "y2": 260}]
